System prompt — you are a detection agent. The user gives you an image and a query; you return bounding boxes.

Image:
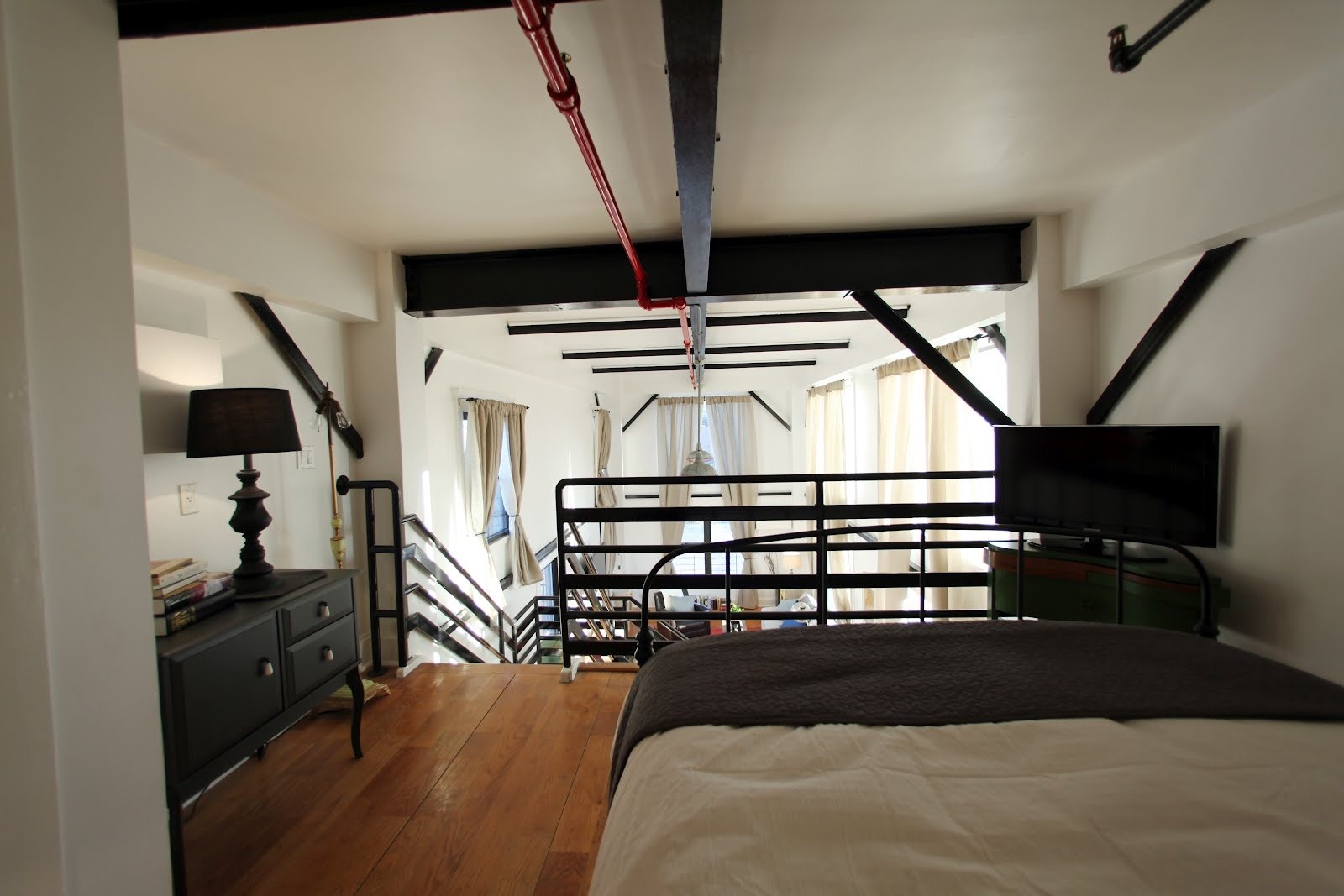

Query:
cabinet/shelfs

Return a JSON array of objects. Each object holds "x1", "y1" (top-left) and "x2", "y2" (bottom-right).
[{"x1": 983, "y1": 542, "x2": 1234, "y2": 640}]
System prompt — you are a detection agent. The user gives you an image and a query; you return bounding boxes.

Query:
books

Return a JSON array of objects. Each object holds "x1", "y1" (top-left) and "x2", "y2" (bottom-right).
[
  {"x1": 150, "y1": 558, "x2": 236, "y2": 637},
  {"x1": 695, "y1": 594, "x2": 733, "y2": 610}
]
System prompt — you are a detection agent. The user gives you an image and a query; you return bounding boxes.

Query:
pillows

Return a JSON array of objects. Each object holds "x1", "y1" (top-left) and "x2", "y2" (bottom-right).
[
  {"x1": 793, "y1": 596, "x2": 815, "y2": 610},
  {"x1": 778, "y1": 618, "x2": 808, "y2": 629},
  {"x1": 667, "y1": 595, "x2": 696, "y2": 612}
]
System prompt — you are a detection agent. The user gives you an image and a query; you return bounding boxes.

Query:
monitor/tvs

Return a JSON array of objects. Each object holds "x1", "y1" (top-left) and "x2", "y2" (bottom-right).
[{"x1": 993, "y1": 424, "x2": 1221, "y2": 563}]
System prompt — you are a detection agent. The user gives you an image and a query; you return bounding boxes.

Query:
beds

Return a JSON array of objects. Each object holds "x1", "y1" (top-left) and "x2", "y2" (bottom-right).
[{"x1": 588, "y1": 620, "x2": 1344, "y2": 896}]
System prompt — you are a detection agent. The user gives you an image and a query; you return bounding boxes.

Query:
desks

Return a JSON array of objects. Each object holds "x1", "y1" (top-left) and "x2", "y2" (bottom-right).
[{"x1": 154, "y1": 569, "x2": 366, "y2": 896}]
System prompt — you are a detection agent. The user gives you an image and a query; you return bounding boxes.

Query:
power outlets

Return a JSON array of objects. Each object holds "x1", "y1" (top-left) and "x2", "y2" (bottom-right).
[{"x1": 179, "y1": 482, "x2": 201, "y2": 514}]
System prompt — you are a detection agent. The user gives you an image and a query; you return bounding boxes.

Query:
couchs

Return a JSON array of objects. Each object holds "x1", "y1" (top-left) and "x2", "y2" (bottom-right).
[
  {"x1": 654, "y1": 591, "x2": 710, "y2": 640},
  {"x1": 761, "y1": 592, "x2": 838, "y2": 630}
]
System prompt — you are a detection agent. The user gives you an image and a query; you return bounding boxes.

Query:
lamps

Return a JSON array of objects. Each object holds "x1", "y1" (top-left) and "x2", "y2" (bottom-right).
[
  {"x1": 685, "y1": 379, "x2": 712, "y2": 461},
  {"x1": 180, "y1": 386, "x2": 298, "y2": 596},
  {"x1": 679, "y1": 376, "x2": 718, "y2": 478},
  {"x1": 790, "y1": 602, "x2": 811, "y2": 626}
]
